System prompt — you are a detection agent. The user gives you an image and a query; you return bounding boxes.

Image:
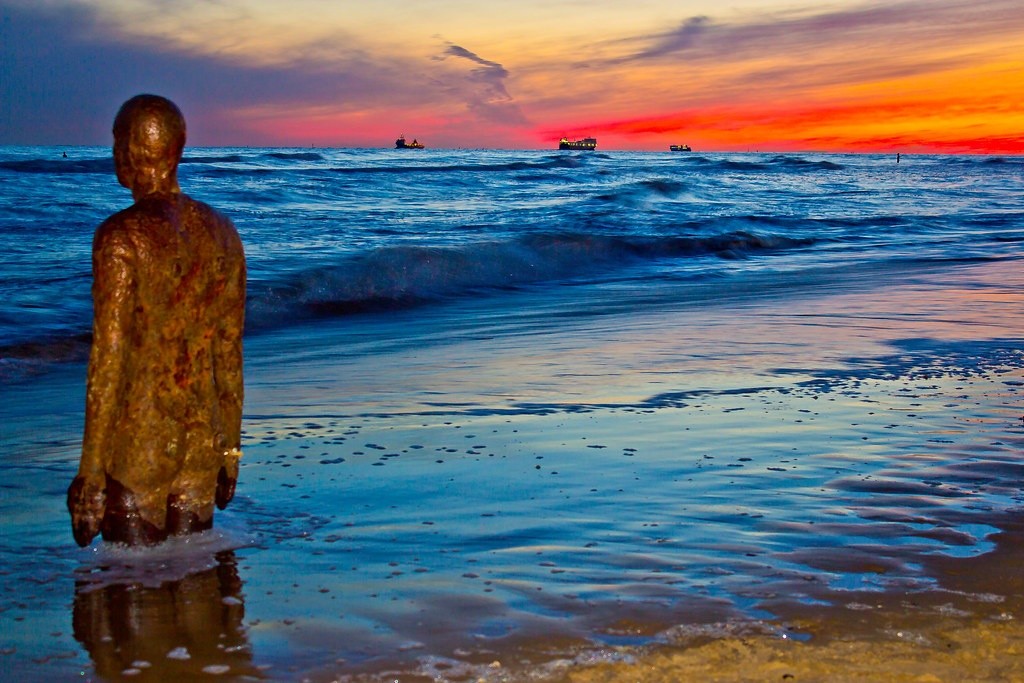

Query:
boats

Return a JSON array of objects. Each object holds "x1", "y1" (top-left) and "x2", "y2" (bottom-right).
[
  {"x1": 394, "y1": 132, "x2": 425, "y2": 150},
  {"x1": 670, "y1": 143, "x2": 692, "y2": 152},
  {"x1": 559, "y1": 137, "x2": 597, "y2": 149}
]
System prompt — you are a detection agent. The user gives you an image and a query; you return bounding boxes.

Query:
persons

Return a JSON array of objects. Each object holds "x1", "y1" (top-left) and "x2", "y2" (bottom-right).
[{"x1": 67, "y1": 94, "x2": 247, "y2": 548}]
